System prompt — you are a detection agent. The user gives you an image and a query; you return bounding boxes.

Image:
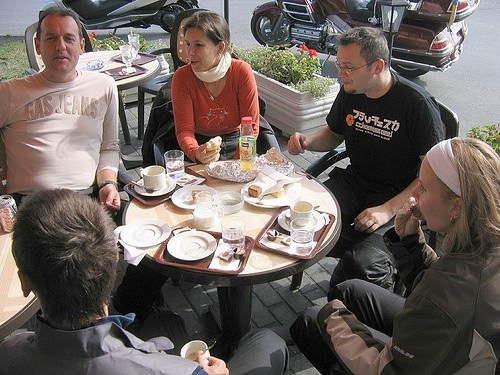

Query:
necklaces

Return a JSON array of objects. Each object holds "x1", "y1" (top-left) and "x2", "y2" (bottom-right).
[{"x1": 209, "y1": 81, "x2": 220, "y2": 101}]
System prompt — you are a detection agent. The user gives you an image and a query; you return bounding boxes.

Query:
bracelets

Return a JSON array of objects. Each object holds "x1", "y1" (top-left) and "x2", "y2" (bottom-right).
[{"x1": 100, "y1": 181, "x2": 118, "y2": 191}]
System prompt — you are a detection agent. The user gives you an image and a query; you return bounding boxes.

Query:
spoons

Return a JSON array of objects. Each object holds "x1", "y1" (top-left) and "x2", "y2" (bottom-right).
[{"x1": 132, "y1": 181, "x2": 154, "y2": 193}]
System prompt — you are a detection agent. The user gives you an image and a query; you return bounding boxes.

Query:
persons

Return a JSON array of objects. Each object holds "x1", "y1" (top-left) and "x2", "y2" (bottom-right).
[
  {"x1": 0, "y1": 189, "x2": 290, "y2": 375},
  {"x1": 0, "y1": 7, "x2": 179, "y2": 325},
  {"x1": 287, "y1": 26, "x2": 445, "y2": 289},
  {"x1": 289, "y1": 137, "x2": 500, "y2": 375},
  {"x1": 171, "y1": 11, "x2": 260, "y2": 165},
  {"x1": 347, "y1": 0, "x2": 376, "y2": 23}
]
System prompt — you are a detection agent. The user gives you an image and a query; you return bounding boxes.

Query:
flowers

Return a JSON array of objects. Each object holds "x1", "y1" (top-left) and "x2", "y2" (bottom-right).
[
  {"x1": 229, "y1": 43, "x2": 338, "y2": 101},
  {"x1": 89, "y1": 28, "x2": 156, "y2": 52}
]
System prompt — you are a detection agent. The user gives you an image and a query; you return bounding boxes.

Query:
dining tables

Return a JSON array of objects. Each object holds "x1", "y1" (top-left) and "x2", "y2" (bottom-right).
[{"x1": 122, "y1": 159, "x2": 341, "y2": 358}]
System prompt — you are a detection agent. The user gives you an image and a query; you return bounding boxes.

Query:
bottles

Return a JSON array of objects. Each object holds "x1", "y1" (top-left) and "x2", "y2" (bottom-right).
[{"x1": 239, "y1": 117, "x2": 257, "y2": 172}]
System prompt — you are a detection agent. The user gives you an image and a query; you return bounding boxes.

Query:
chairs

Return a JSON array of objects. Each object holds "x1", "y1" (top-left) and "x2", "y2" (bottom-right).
[
  {"x1": 138, "y1": 9, "x2": 211, "y2": 139},
  {"x1": 25, "y1": 19, "x2": 131, "y2": 147},
  {"x1": 290, "y1": 95, "x2": 461, "y2": 294},
  {"x1": 142, "y1": 75, "x2": 280, "y2": 170}
]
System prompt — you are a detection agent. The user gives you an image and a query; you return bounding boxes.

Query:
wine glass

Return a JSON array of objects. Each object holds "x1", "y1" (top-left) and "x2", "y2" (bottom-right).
[
  {"x1": 119, "y1": 45, "x2": 136, "y2": 74},
  {"x1": 127, "y1": 34, "x2": 141, "y2": 60}
]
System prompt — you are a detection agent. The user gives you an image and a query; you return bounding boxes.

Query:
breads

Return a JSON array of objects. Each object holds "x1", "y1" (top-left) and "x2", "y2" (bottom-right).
[
  {"x1": 248, "y1": 185, "x2": 262, "y2": 197},
  {"x1": 191, "y1": 189, "x2": 208, "y2": 203},
  {"x1": 205, "y1": 136, "x2": 222, "y2": 152},
  {"x1": 271, "y1": 187, "x2": 285, "y2": 197}
]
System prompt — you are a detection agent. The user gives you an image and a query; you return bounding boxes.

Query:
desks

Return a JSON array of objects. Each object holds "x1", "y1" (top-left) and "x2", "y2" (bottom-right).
[{"x1": 76, "y1": 50, "x2": 161, "y2": 145}]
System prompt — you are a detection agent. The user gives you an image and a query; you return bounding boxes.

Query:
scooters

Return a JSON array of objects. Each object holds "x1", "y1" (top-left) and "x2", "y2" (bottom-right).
[
  {"x1": 38, "y1": 0, "x2": 202, "y2": 35},
  {"x1": 250, "y1": 0, "x2": 481, "y2": 78}
]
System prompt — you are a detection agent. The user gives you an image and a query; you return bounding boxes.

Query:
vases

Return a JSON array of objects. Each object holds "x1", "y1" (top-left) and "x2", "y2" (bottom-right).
[
  {"x1": 124, "y1": 55, "x2": 171, "y2": 104},
  {"x1": 252, "y1": 69, "x2": 340, "y2": 137}
]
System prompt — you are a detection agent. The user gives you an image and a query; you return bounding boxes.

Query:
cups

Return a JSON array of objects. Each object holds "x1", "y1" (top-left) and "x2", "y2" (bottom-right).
[
  {"x1": 290, "y1": 201, "x2": 313, "y2": 226},
  {"x1": 164, "y1": 150, "x2": 184, "y2": 179},
  {"x1": 290, "y1": 218, "x2": 315, "y2": 256},
  {"x1": 193, "y1": 192, "x2": 223, "y2": 229},
  {"x1": 221, "y1": 219, "x2": 246, "y2": 254},
  {"x1": 180, "y1": 340, "x2": 210, "y2": 365},
  {"x1": 140, "y1": 166, "x2": 166, "y2": 190}
]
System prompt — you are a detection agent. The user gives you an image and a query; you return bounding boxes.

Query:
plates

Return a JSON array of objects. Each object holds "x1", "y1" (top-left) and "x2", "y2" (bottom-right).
[
  {"x1": 278, "y1": 209, "x2": 325, "y2": 232},
  {"x1": 171, "y1": 185, "x2": 216, "y2": 209},
  {"x1": 241, "y1": 181, "x2": 300, "y2": 208},
  {"x1": 134, "y1": 177, "x2": 176, "y2": 196},
  {"x1": 120, "y1": 217, "x2": 171, "y2": 247},
  {"x1": 167, "y1": 230, "x2": 217, "y2": 261}
]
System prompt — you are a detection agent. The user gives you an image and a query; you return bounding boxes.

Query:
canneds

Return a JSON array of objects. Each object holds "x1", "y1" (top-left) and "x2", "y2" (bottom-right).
[{"x1": 0, "y1": 195, "x2": 17, "y2": 233}]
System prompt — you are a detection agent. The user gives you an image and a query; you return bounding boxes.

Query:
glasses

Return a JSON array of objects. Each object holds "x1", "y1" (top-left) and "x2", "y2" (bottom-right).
[{"x1": 334, "y1": 60, "x2": 388, "y2": 74}]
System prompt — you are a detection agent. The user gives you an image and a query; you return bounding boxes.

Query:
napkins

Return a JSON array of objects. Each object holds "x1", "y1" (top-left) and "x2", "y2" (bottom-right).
[
  {"x1": 169, "y1": 171, "x2": 205, "y2": 188},
  {"x1": 208, "y1": 237, "x2": 240, "y2": 271},
  {"x1": 114, "y1": 225, "x2": 151, "y2": 266},
  {"x1": 260, "y1": 229, "x2": 317, "y2": 256},
  {"x1": 246, "y1": 164, "x2": 304, "y2": 207}
]
